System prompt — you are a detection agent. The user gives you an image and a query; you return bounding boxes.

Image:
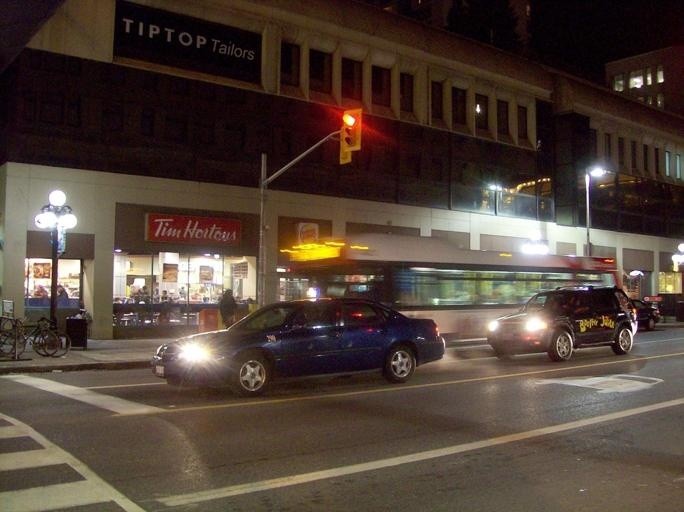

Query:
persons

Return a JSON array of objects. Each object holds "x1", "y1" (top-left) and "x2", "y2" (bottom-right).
[
  {"x1": 55, "y1": 285, "x2": 69, "y2": 306},
  {"x1": 220, "y1": 288, "x2": 236, "y2": 328},
  {"x1": 130, "y1": 285, "x2": 187, "y2": 303},
  {"x1": 33, "y1": 284, "x2": 48, "y2": 297}
]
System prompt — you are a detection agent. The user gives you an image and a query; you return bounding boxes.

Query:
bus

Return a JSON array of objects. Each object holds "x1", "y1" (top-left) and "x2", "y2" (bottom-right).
[{"x1": 284, "y1": 232, "x2": 622, "y2": 336}]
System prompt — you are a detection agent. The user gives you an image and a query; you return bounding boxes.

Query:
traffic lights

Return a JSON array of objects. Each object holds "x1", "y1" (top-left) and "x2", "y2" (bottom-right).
[{"x1": 343, "y1": 108, "x2": 361, "y2": 151}]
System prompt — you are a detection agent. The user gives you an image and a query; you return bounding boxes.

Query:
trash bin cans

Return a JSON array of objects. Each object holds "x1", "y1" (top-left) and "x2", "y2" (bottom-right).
[{"x1": 64, "y1": 316, "x2": 87, "y2": 351}]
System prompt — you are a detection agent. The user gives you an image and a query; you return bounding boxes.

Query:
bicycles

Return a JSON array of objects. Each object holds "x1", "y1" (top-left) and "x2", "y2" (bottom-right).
[{"x1": 0, "y1": 315, "x2": 71, "y2": 357}]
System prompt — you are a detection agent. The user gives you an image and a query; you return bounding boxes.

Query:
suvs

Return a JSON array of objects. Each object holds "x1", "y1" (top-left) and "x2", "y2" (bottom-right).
[{"x1": 486, "y1": 285, "x2": 638, "y2": 363}]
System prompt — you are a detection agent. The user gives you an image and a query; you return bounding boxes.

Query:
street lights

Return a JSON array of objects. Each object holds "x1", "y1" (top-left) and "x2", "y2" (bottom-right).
[
  {"x1": 585, "y1": 166, "x2": 605, "y2": 256},
  {"x1": 671, "y1": 243, "x2": 684, "y2": 301},
  {"x1": 34, "y1": 189, "x2": 77, "y2": 349}
]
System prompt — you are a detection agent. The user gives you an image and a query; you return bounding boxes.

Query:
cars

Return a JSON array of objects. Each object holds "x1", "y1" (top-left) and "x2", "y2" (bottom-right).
[
  {"x1": 150, "y1": 295, "x2": 445, "y2": 399},
  {"x1": 629, "y1": 299, "x2": 661, "y2": 331}
]
